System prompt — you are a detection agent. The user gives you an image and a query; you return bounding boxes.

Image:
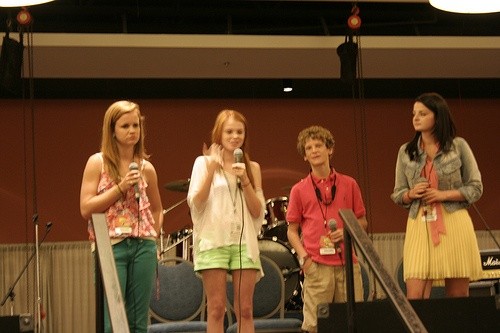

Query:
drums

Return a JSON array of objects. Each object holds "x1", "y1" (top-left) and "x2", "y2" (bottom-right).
[
  {"x1": 263, "y1": 195, "x2": 288, "y2": 235},
  {"x1": 253, "y1": 236, "x2": 303, "y2": 312}
]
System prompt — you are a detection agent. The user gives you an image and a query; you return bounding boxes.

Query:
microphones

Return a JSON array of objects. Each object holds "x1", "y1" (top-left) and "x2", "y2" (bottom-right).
[
  {"x1": 329, "y1": 219, "x2": 342, "y2": 254},
  {"x1": 233, "y1": 148, "x2": 243, "y2": 187},
  {"x1": 128, "y1": 162, "x2": 140, "y2": 201},
  {"x1": 416, "y1": 177, "x2": 428, "y2": 215}
]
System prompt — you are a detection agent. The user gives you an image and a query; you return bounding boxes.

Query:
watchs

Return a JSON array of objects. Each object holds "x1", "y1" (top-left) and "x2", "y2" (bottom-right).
[{"x1": 299, "y1": 253, "x2": 311, "y2": 265}]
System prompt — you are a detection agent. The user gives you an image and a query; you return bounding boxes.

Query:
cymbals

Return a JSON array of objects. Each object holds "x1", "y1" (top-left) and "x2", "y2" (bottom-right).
[{"x1": 165, "y1": 179, "x2": 189, "y2": 192}]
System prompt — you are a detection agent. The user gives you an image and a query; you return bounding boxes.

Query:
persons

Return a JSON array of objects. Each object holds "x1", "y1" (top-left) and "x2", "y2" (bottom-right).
[
  {"x1": 390, "y1": 92, "x2": 484, "y2": 302},
  {"x1": 284, "y1": 124, "x2": 369, "y2": 333},
  {"x1": 79, "y1": 100, "x2": 165, "y2": 333},
  {"x1": 186, "y1": 110, "x2": 268, "y2": 333}
]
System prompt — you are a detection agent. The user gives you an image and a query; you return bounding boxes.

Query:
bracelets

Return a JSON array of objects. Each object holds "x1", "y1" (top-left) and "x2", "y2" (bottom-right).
[
  {"x1": 113, "y1": 183, "x2": 124, "y2": 198},
  {"x1": 408, "y1": 188, "x2": 413, "y2": 202},
  {"x1": 241, "y1": 180, "x2": 252, "y2": 187}
]
